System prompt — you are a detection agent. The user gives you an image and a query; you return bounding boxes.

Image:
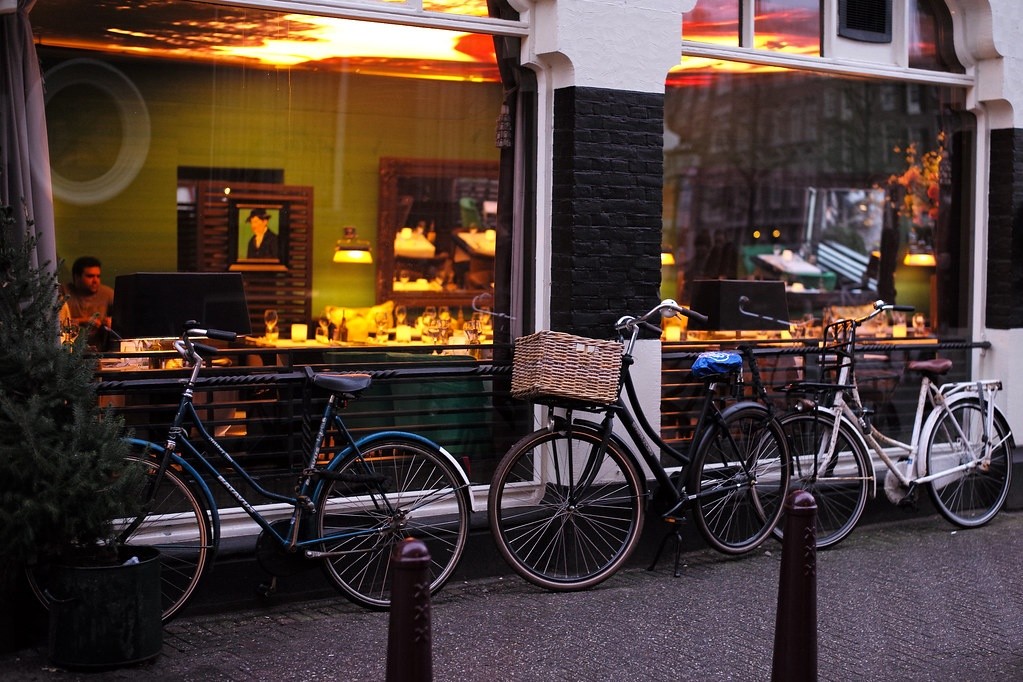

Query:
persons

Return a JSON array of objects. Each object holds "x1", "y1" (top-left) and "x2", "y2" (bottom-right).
[{"x1": 60, "y1": 256, "x2": 114, "y2": 329}]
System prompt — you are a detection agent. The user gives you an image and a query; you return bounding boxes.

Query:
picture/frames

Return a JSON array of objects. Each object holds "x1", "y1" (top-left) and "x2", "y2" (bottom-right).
[{"x1": 221, "y1": 198, "x2": 291, "y2": 273}]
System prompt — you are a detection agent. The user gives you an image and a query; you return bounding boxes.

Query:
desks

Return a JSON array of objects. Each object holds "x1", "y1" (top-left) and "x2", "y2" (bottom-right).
[{"x1": 247, "y1": 324, "x2": 501, "y2": 368}]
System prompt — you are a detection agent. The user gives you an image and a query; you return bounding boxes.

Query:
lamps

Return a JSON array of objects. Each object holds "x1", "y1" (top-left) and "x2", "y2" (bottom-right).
[{"x1": 904, "y1": 221, "x2": 938, "y2": 267}]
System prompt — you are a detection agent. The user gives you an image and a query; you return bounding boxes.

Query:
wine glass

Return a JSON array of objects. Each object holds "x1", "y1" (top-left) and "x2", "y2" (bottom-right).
[
  {"x1": 395, "y1": 305, "x2": 407, "y2": 325},
  {"x1": 263, "y1": 309, "x2": 278, "y2": 346},
  {"x1": 422, "y1": 305, "x2": 451, "y2": 333},
  {"x1": 374, "y1": 311, "x2": 387, "y2": 342},
  {"x1": 463, "y1": 319, "x2": 482, "y2": 343}
]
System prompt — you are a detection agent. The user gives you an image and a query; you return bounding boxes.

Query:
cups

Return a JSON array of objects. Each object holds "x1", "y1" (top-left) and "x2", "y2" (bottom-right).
[
  {"x1": 315, "y1": 327, "x2": 325, "y2": 342},
  {"x1": 291, "y1": 323, "x2": 308, "y2": 342}
]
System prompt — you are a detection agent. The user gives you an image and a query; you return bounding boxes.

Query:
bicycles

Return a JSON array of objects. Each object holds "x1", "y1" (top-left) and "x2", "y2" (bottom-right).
[
  {"x1": 747, "y1": 300, "x2": 1017, "y2": 553},
  {"x1": 11, "y1": 319, "x2": 478, "y2": 640},
  {"x1": 488, "y1": 298, "x2": 796, "y2": 594}
]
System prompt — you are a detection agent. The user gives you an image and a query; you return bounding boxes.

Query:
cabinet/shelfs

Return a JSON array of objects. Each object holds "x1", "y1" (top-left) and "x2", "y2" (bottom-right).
[{"x1": 370, "y1": 151, "x2": 501, "y2": 319}]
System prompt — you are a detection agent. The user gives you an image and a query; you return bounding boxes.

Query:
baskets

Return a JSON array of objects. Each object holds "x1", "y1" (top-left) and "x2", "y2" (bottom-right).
[{"x1": 510, "y1": 329, "x2": 624, "y2": 406}]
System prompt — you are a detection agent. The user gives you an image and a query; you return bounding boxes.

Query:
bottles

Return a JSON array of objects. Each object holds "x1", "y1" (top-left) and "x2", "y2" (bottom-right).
[
  {"x1": 149, "y1": 340, "x2": 164, "y2": 370},
  {"x1": 338, "y1": 317, "x2": 348, "y2": 342}
]
